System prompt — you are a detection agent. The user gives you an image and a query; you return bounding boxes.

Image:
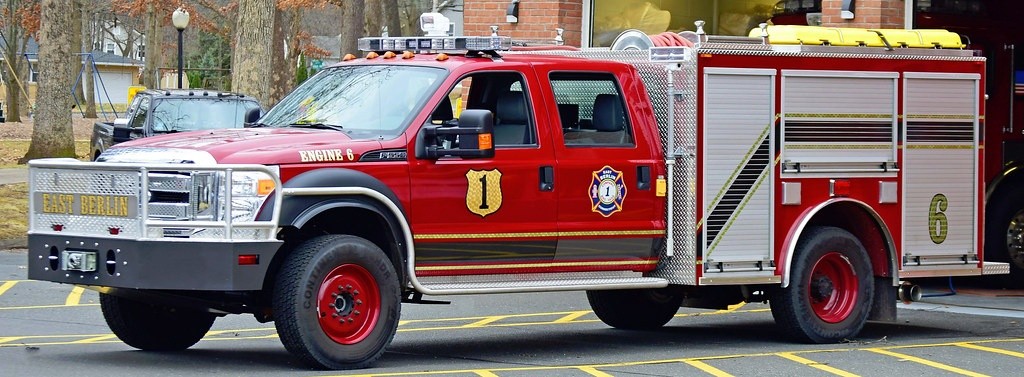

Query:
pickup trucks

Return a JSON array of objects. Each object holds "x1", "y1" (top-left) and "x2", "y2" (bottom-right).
[{"x1": 90, "y1": 87, "x2": 264, "y2": 162}]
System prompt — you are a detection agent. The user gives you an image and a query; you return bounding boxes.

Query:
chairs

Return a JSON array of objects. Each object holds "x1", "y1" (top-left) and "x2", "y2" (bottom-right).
[
  {"x1": 590, "y1": 94, "x2": 629, "y2": 145},
  {"x1": 493, "y1": 90, "x2": 531, "y2": 145}
]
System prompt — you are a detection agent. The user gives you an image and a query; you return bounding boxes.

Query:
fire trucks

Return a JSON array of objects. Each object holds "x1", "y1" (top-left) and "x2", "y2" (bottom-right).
[
  {"x1": 27, "y1": 11, "x2": 1012, "y2": 370},
  {"x1": 912, "y1": 1, "x2": 1024, "y2": 289}
]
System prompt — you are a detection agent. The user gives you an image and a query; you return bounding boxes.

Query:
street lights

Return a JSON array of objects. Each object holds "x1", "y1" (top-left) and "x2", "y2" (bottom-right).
[{"x1": 171, "y1": 6, "x2": 189, "y2": 90}]
